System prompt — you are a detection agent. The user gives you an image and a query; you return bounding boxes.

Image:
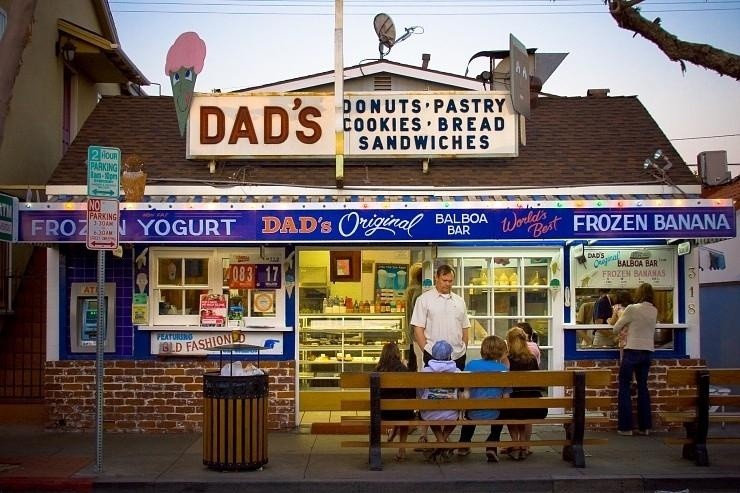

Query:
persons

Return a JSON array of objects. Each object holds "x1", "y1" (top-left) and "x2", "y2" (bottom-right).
[
  {"x1": 606, "y1": 290, "x2": 629, "y2": 363},
  {"x1": 610, "y1": 282, "x2": 658, "y2": 437},
  {"x1": 574, "y1": 289, "x2": 607, "y2": 348},
  {"x1": 411, "y1": 263, "x2": 470, "y2": 373},
  {"x1": 459, "y1": 336, "x2": 514, "y2": 463},
  {"x1": 402, "y1": 263, "x2": 422, "y2": 372},
  {"x1": 512, "y1": 323, "x2": 540, "y2": 370},
  {"x1": 371, "y1": 343, "x2": 416, "y2": 462},
  {"x1": 416, "y1": 340, "x2": 465, "y2": 464},
  {"x1": 594, "y1": 286, "x2": 616, "y2": 346},
  {"x1": 501, "y1": 329, "x2": 549, "y2": 460}
]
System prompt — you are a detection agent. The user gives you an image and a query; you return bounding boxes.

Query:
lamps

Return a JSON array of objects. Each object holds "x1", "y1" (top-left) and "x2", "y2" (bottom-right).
[{"x1": 643, "y1": 149, "x2": 688, "y2": 198}]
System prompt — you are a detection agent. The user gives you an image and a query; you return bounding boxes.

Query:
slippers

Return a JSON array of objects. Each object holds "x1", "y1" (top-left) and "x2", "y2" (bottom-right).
[
  {"x1": 395, "y1": 453, "x2": 407, "y2": 461},
  {"x1": 387, "y1": 428, "x2": 393, "y2": 442}
]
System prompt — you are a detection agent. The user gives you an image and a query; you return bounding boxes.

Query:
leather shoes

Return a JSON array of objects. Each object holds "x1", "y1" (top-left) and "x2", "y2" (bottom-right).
[
  {"x1": 617, "y1": 430, "x2": 632, "y2": 435},
  {"x1": 634, "y1": 429, "x2": 649, "y2": 435}
]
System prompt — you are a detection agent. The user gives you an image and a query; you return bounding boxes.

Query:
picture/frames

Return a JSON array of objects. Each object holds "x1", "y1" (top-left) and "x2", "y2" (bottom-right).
[
  {"x1": 374, "y1": 263, "x2": 409, "y2": 305},
  {"x1": 333, "y1": 255, "x2": 353, "y2": 279}
]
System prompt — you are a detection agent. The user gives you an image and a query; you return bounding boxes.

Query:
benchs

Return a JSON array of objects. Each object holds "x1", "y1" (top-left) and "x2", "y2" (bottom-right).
[
  {"x1": 658, "y1": 368, "x2": 740, "y2": 466},
  {"x1": 340, "y1": 369, "x2": 612, "y2": 471}
]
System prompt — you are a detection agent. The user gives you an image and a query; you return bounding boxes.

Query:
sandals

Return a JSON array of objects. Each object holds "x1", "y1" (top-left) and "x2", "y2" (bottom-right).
[
  {"x1": 508, "y1": 448, "x2": 520, "y2": 459},
  {"x1": 520, "y1": 448, "x2": 526, "y2": 458}
]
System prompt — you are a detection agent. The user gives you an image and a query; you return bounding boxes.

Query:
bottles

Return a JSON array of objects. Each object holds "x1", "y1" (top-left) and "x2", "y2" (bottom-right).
[
  {"x1": 481, "y1": 273, "x2": 487, "y2": 290},
  {"x1": 353, "y1": 300, "x2": 358, "y2": 312},
  {"x1": 401, "y1": 300, "x2": 405, "y2": 312},
  {"x1": 528, "y1": 271, "x2": 547, "y2": 292},
  {"x1": 494, "y1": 271, "x2": 499, "y2": 291},
  {"x1": 364, "y1": 300, "x2": 370, "y2": 313},
  {"x1": 358, "y1": 301, "x2": 364, "y2": 312},
  {"x1": 339, "y1": 296, "x2": 344, "y2": 306},
  {"x1": 470, "y1": 278, "x2": 481, "y2": 294},
  {"x1": 323, "y1": 298, "x2": 327, "y2": 313},
  {"x1": 498, "y1": 272, "x2": 509, "y2": 291},
  {"x1": 509, "y1": 273, "x2": 518, "y2": 291},
  {"x1": 375, "y1": 300, "x2": 381, "y2": 313},
  {"x1": 347, "y1": 298, "x2": 354, "y2": 312},
  {"x1": 396, "y1": 300, "x2": 401, "y2": 313},
  {"x1": 333, "y1": 294, "x2": 339, "y2": 306},
  {"x1": 391, "y1": 301, "x2": 396, "y2": 313},
  {"x1": 299, "y1": 303, "x2": 313, "y2": 313},
  {"x1": 312, "y1": 304, "x2": 318, "y2": 313},
  {"x1": 385, "y1": 302, "x2": 390, "y2": 312},
  {"x1": 370, "y1": 300, "x2": 375, "y2": 314},
  {"x1": 381, "y1": 302, "x2": 386, "y2": 313},
  {"x1": 317, "y1": 305, "x2": 320, "y2": 313},
  {"x1": 328, "y1": 296, "x2": 333, "y2": 307}
]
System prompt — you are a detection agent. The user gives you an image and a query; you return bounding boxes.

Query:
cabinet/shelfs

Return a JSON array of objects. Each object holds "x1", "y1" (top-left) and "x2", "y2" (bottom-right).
[
  {"x1": 531, "y1": 329, "x2": 548, "y2": 369},
  {"x1": 299, "y1": 286, "x2": 330, "y2": 314},
  {"x1": 299, "y1": 315, "x2": 405, "y2": 411},
  {"x1": 434, "y1": 266, "x2": 548, "y2": 339}
]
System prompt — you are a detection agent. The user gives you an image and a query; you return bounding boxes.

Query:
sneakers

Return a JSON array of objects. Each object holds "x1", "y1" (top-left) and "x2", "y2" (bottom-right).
[
  {"x1": 439, "y1": 442, "x2": 450, "y2": 462},
  {"x1": 415, "y1": 436, "x2": 428, "y2": 451},
  {"x1": 487, "y1": 450, "x2": 500, "y2": 462},
  {"x1": 429, "y1": 446, "x2": 441, "y2": 463},
  {"x1": 459, "y1": 447, "x2": 472, "y2": 455}
]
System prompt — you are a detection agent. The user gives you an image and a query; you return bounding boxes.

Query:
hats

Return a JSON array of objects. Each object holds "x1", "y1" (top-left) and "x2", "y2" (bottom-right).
[{"x1": 432, "y1": 341, "x2": 452, "y2": 359}]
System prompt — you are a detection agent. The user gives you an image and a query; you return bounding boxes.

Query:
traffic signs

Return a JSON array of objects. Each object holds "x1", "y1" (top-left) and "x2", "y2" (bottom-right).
[
  {"x1": 87, "y1": 146, "x2": 121, "y2": 198},
  {"x1": 87, "y1": 200, "x2": 118, "y2": 248}
]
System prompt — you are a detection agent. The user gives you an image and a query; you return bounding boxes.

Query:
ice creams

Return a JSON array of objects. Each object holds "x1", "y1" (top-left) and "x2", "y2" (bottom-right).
[
  {"x1": 135, "y1": 272, "x2": 147, "y2": 293},
  {"x1": 165, "y1": 31, "x2": 206, "y2": 138}
]
